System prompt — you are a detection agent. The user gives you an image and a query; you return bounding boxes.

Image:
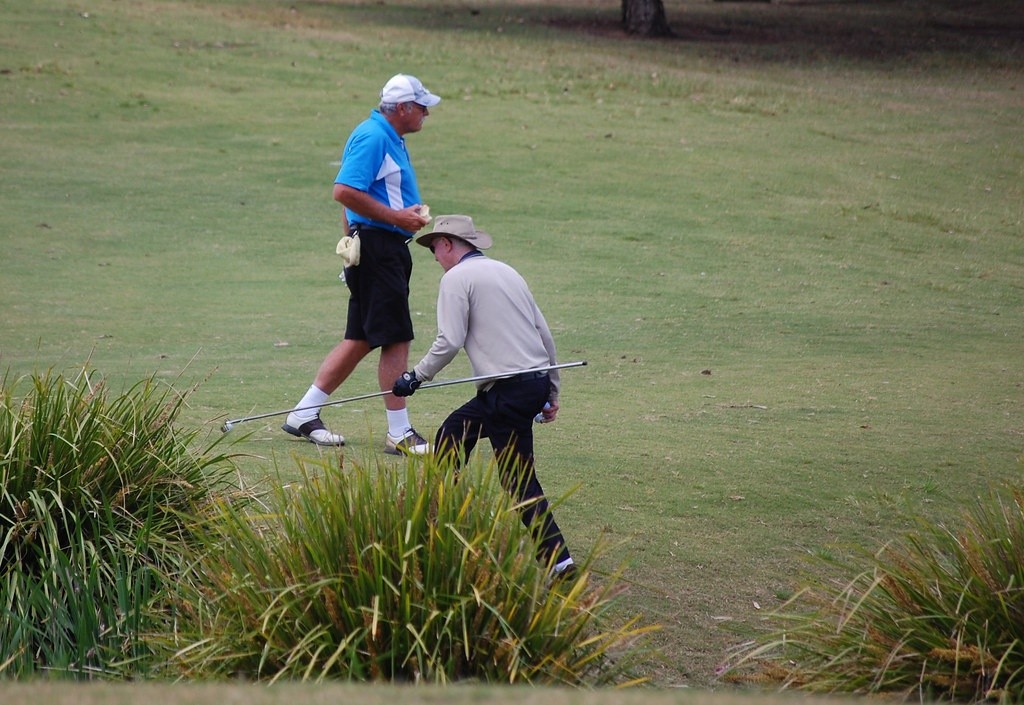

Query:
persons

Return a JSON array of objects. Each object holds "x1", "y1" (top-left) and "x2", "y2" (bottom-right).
[
  {"x1": 281, "y1": 72, "x2": 441, "y2": 458},
  {"x1": 390, "y1": 214, "x2": 580, "y2": 582}
]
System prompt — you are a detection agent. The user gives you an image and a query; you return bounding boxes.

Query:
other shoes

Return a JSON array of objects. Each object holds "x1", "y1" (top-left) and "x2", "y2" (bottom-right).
[{"x1": 544, "y1": 562, "x2": 578, "y2": 589}]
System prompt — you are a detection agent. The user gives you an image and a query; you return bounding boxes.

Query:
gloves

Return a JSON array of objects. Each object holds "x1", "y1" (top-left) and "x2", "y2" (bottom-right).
[
  {"x1": 392, "y1": 369, "x2": 422, "y2": 396},
  {"x1": 339, "y1": 272, "x2": 347, "y2": 287}
]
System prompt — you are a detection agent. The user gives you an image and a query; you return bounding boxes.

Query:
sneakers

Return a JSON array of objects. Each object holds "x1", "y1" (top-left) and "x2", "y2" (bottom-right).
[
  {"x1": 280, "y1": 412, "x2": 345, "y2": 446},
  {"x1": 385, "y1": 424, "x2": 434, "y2": 457}
]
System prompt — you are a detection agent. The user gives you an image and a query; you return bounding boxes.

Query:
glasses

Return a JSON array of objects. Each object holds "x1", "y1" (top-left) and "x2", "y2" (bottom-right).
[{"x1": 429, "y1": 237, "x2": 442, "y2": 254}]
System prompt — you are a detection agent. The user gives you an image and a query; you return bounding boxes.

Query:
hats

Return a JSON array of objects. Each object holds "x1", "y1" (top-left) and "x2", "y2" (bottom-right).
[
  {"x1": 416, "y1": 214, "x2": 493, "y2": 250},
  {"x1": 380, "y1": 72, "x2": 441, "y2": 106}
]
